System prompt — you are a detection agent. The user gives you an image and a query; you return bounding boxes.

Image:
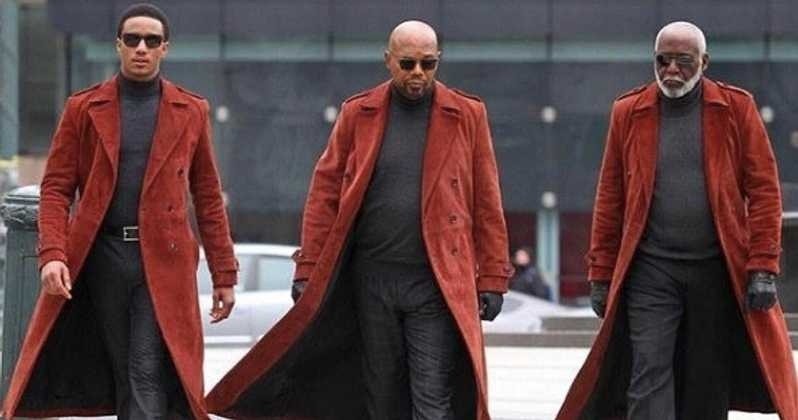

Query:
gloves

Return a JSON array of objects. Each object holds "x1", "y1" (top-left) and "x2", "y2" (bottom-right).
[
  {"x1": 744, "y1": 272, "x2": 779, "y2": 314},
  {"x1": 478, "y1": 292, "x2": 503, "y2": 322},
  {"x1": 588, "y1": 281, "x2": 610, "y2": 319},
  {"x1": 292, "y1": 281, "x2": 307, "y2": 302}
]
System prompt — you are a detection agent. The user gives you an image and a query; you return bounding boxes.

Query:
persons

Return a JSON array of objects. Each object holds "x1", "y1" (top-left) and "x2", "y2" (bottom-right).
[
  {"x1": 202, "y1": 20, "x2": 512, "y2": 420},
  {"x1": 507, "y1": 249, "x2": 545, "y2": 300},
  {"x1": 554, "y1": 21, "x2": 798, "y2": 420},
  {"x1": 3, "y1": 2, "x2": 240, "y2": 420}
]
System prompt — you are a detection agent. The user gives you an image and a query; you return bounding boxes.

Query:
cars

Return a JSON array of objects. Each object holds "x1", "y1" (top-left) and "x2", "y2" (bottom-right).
[{"x1": 196, "y1": 243, "x2": 303, "y2": 346}]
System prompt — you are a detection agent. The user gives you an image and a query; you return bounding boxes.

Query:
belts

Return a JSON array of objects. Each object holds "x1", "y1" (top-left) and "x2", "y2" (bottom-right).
[{"x1": 103, "y1": 224, "x2": 140, "y2": 241}]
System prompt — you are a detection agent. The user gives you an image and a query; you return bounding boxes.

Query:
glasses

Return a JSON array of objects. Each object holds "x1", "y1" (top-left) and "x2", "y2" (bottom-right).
[
  {"x1": 399, "y1": 55, "x2": 437, "y2": 71},
  {"x1": 654, "y1": 53, "x2": 694, "y2": 65},
  {"x1": 122, "y1": 32, "x2": 167, "y2": 48}
]
